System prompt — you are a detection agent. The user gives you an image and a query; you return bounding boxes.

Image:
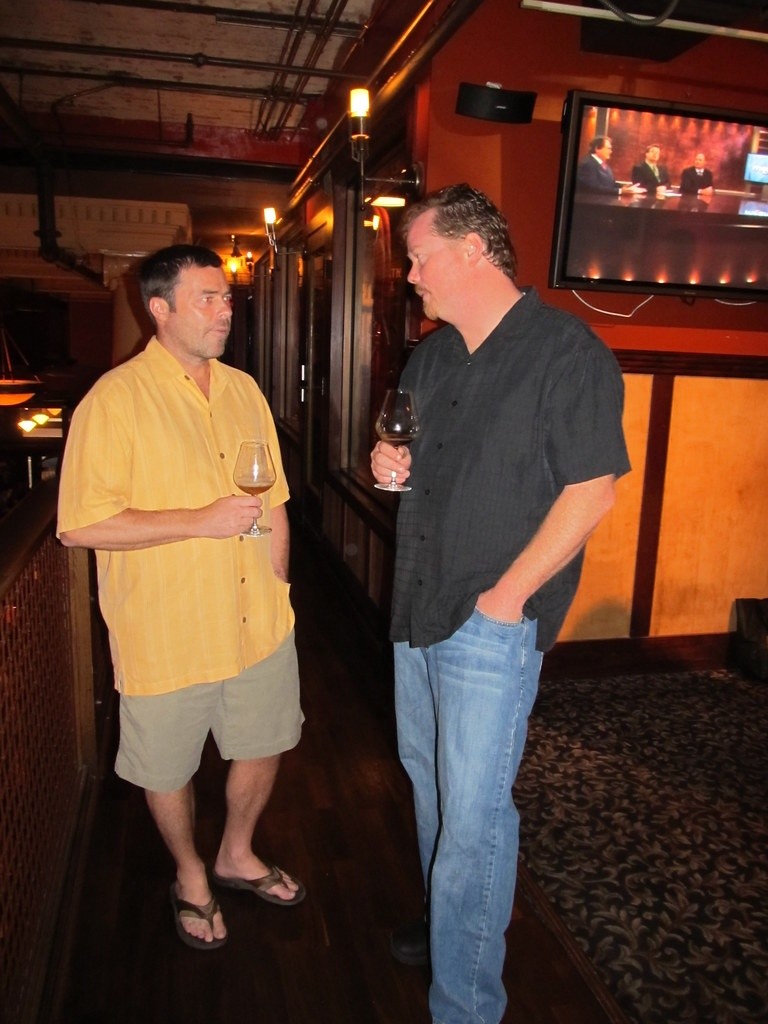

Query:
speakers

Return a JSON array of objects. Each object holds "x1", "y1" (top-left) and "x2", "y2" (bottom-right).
[{"x1": 456, "y1": 83, "x2": 538, "y2": 124}]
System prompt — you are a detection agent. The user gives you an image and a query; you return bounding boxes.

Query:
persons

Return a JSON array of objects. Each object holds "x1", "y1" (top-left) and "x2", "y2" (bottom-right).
[
  {"x1": 55, "y1": 242, "x2": 307, "y2": 951},
  {"x1": 573, "y1": 135, "x2": 713, "y2": 195},
  {"x1": 369, "y1": 180, "x2": 633, "y2": 1023}
]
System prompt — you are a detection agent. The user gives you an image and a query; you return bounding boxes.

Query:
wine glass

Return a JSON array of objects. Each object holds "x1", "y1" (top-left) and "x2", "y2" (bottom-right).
[
  {"x1": 374, "y1": 390, "x2": 420, "y2": 492},
  {"x1": 233, "y1": 442, "x2": 276, "y2": 537}
]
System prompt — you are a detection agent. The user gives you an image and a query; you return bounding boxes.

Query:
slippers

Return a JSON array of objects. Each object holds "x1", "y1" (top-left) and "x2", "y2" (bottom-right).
[
  {"x1": 211, "y1": 864, "x2": 307, "y2": 905},
  {"x1": 169, "y1": 881, "x2": 229, "y2": 949}
]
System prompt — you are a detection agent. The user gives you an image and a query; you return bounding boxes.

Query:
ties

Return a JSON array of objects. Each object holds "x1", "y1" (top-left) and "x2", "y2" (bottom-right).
[
  {"x1": 652, "y1": 165, "x2": 659, "y2": 181},
  {"x1": 602, "y1": 162, "x2": 607, "y2": 170},
  {"x1": 698, "y1": 171, "x2": 702, "y2": 176}
]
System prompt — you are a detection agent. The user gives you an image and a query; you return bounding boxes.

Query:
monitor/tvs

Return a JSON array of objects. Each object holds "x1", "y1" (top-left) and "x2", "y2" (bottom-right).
[
  {"x1": 743, "y1": 153, "x2": 768, "y2": 185},
  {"x1": 547, "y1": 90, "x2": 768, "y2": 303}
]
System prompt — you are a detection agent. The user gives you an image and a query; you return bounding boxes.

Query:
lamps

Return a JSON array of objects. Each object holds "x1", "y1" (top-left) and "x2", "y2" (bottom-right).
[
  {"x1": 347, "y1": 86, "x2": 425, "y2": 211},
  {"x1": 262, "y1": 206, "x2": 301, "y2": 261},
  {"x1": 245, "y1": 250, "x2": 274, "y2": 281}
]
